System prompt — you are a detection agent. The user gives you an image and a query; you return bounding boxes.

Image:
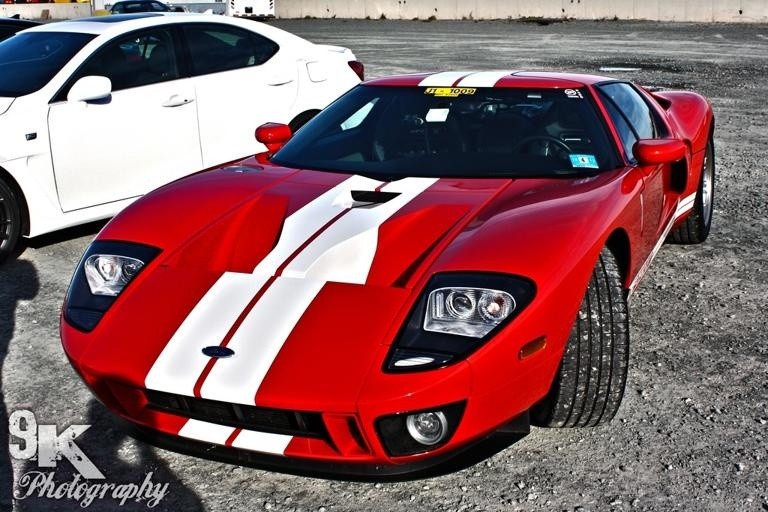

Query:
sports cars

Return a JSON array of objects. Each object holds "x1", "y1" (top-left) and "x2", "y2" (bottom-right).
[{"x1": 61, "y1": 70, "x2": 716, "y2": 481}]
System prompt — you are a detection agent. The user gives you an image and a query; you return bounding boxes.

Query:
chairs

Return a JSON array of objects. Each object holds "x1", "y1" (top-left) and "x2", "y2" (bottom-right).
[{"x1": 368, "y1": 99, "x2": 588, "y2": 176}]
